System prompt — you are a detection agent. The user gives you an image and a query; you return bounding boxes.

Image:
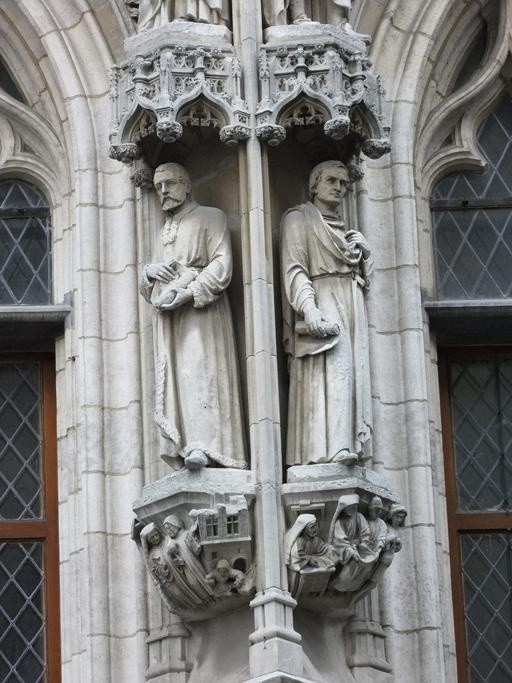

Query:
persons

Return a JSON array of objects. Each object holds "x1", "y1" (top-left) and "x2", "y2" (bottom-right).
[
  {"x1": 139, "y1": 157, "x2": 252, "y2": 468},
  {"x1": 261, "y1": 0, "x2": 373, "y2": 44},
  {"x1": 140, "y1": 492, "x2": 407, "y2": 609},
  {"x1": 276, "y1": 159, "x2": 376, "y2": 469}
]
